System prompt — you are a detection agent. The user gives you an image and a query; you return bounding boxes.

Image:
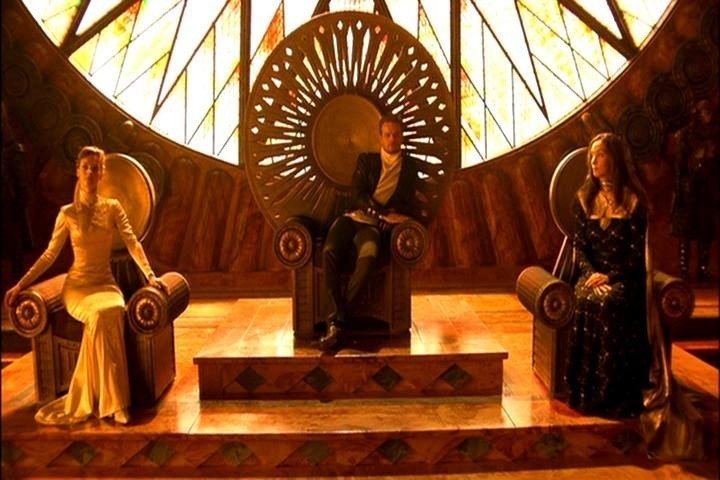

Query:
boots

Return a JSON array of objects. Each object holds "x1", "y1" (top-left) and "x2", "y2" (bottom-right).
[
  {"x1": 679, "y1": 244, "x2": 690, "y2": 278},
  {"x1": 696, "y1": 248, "x2": 714, "y2": 281}
]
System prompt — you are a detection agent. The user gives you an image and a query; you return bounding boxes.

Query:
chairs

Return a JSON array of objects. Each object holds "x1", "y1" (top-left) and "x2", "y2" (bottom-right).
[
  {"x1": 516, "y1": 146, "x2": 695, "y2": 398},
  {"x1": 10, "y1": 153, "x2": 190, "y2": 406},
  {"x1": 240, "y1": 12, "x2": 457, "y2": 345}
]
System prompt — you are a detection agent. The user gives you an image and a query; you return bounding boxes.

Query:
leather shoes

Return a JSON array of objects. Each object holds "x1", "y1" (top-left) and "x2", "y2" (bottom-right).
[
  {"x1": 320, "y1": 322, "x2": 343, "y2": 349},
  {"x1": 113, "y1": 407, "x2": 130, "y2": 423}
]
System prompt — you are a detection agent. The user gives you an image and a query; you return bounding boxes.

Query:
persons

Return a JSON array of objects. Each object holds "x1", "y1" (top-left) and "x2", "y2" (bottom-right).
[
  {"x1": 4, "y1": 146, "x2": 167, "y2": 427},
  {"x1": 315, "y1": 116, "x2": 418, "y2": 353},
  {"x1": 562, "y1": 133, "x2": 649, "y2": 415},
  {"x1": 669, "y1": 99, "x2": 719, "y2": 280}
]
